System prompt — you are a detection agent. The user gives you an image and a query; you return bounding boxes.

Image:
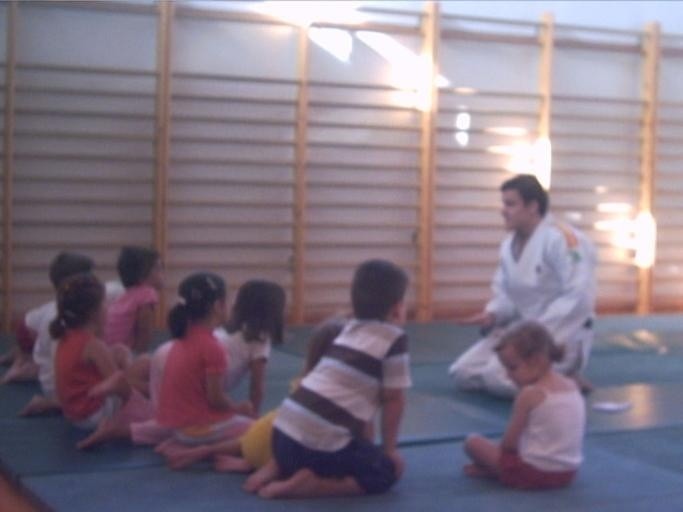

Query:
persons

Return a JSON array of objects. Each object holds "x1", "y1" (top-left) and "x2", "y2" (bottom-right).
[
  {"x1": 72, "y1": 278, "x2": 285, "y2": 445},
  {"x1": 447, "y1": 175, "x2": 595, "y2": 399},
  {"x1": 215, "y1": 315, "x2": 350, "y2": 469},
  {"x1": 1, "y1": 246, "x2": 164, "y2": 424},
  {"x1": 0, "y1": 478, "x2": 34, "y2": 511},
  {"x1": 241, "y1": 263, "x2": 412, "y2": 499},
  {"x1": 462, "y1": 320, "x2": 587, "y2": 489},
  {"x1": 155, "y1": 273, "x2": 258, "y2": 468}
]
